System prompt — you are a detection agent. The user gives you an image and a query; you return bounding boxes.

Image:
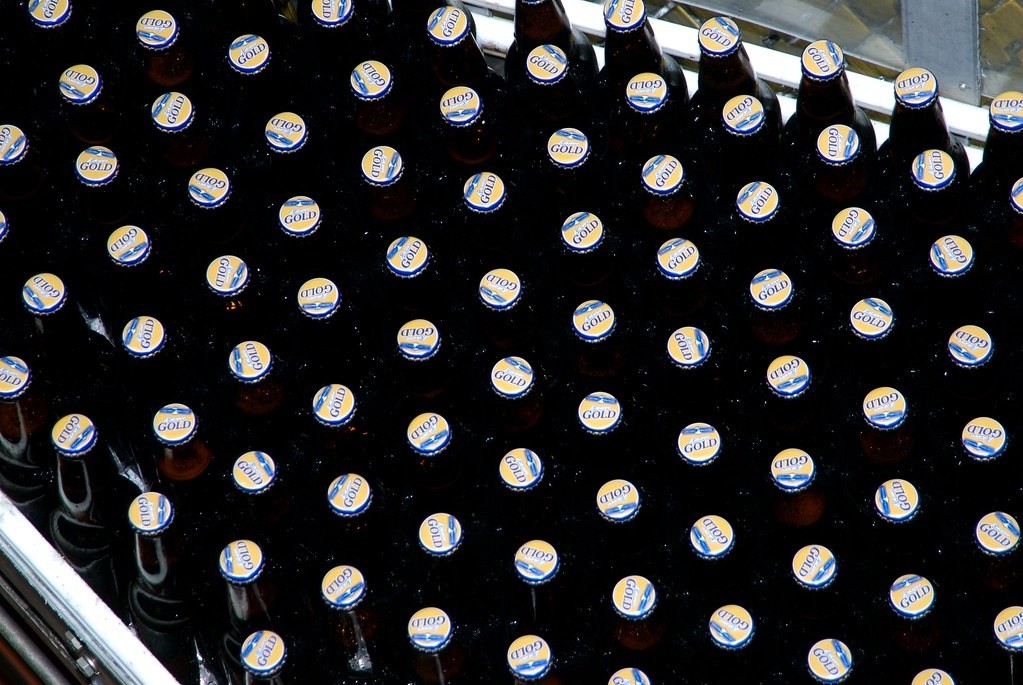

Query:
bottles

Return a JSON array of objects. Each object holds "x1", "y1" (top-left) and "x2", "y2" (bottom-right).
[{"x1": 0, "y1": 0, "x2": 1023, "y2": 685}]
